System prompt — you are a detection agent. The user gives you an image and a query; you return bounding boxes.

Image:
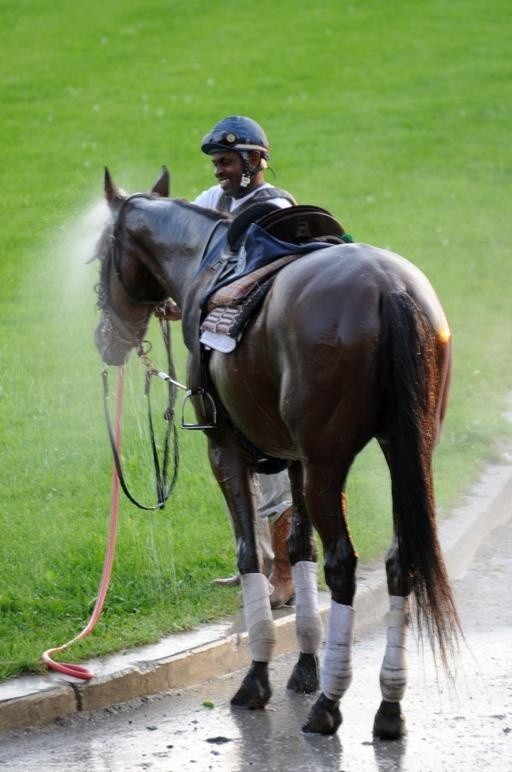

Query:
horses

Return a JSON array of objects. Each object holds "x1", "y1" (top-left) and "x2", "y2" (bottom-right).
[{"x1": 84, "y1": 164, "x2": 467, "y2": 743}]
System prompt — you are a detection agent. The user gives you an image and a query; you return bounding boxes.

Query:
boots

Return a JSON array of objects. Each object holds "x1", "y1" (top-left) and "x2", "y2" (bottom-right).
[{"x1": 213, "y1": 505, "x2": 295, "y2": 609}]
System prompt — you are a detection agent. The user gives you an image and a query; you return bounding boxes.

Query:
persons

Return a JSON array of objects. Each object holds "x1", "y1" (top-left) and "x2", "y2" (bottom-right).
[{"x1": 149, "y1": 116, "x2": 301, "y2": 613}]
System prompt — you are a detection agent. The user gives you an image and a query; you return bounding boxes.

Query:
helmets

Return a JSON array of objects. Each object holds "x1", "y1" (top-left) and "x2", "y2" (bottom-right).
[{"x1": 201, "y1": 115, "x2": 269, "y2": 160}]
